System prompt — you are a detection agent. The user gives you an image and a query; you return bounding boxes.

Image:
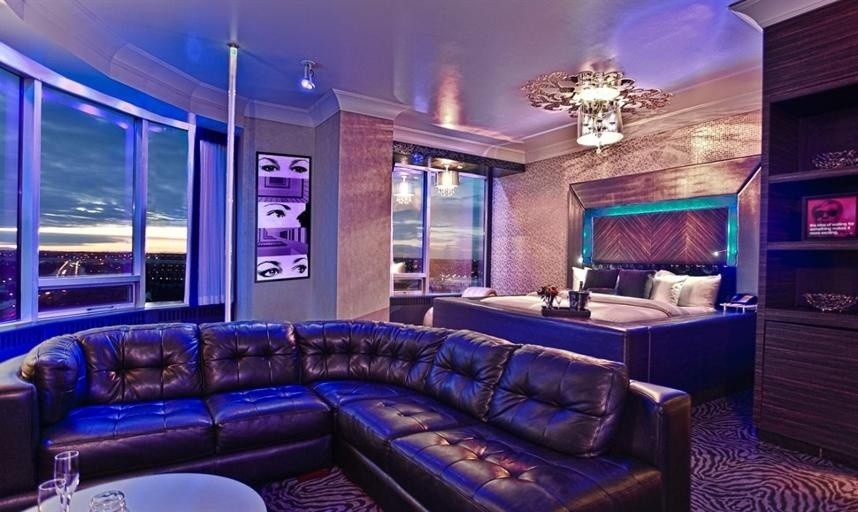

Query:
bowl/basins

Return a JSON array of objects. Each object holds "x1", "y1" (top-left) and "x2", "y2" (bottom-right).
[{"x1": 803, "y1": 293, "x2": 855, "y2": 312}]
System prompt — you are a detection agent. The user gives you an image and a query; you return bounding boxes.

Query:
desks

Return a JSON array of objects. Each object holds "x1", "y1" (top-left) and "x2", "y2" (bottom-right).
[{"x1": 719, "y1": 301, "x2": 757, "y2": 316}]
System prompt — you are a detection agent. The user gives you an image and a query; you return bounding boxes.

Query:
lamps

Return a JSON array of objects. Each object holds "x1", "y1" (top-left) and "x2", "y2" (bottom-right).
[
  {"x1": 524, "y1": 62, "x2": 673, "y2": 156},
  {"x1": 298, "y1": 60, "x2": 317, "y2": 90},
  {"x1": 393, "y1": 170, "x2": 417, "y2": 204},
  {"x1": 433, "y1": 159, "x2": 461, "y2": 198}
]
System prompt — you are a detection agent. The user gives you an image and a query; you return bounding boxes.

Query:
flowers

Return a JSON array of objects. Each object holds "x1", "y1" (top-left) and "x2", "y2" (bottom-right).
[{"x1": 536, "y1": 282, "x2": 559, "y2": 303}]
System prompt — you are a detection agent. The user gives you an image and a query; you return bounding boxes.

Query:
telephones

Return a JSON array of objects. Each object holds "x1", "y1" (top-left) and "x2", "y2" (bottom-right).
[{"x1": 731, "y1": 293, "x2": 758, "y2": 305}]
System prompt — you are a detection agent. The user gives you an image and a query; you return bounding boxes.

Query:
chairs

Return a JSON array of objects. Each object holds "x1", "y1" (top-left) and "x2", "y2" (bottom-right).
[{"x1": 422, "y1": 282, "x2": 497, "y2": 329}]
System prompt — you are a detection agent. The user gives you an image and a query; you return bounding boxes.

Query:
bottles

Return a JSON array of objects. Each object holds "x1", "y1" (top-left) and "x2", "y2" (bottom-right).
[
  {"x1": 88, "y1": 489, "x2": 129, "y2": 512},
  {"x1": 576, "y1": 280, "x2": 588, "y2": 312}
]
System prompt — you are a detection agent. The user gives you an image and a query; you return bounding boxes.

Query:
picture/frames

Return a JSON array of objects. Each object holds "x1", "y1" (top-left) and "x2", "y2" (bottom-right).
[{"x1": 254, "y1": 149, "x2": 313, "y2": 283}]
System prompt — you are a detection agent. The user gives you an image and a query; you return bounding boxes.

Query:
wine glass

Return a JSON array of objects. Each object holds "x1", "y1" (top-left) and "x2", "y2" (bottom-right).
[{"x1": 53, "y1": 451, "x2": 82, "y2": 511}]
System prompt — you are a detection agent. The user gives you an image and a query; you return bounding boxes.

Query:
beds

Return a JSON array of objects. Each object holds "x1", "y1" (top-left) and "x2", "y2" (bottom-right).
[
  {"x1": 422, "y1": 259, "x2": 757, "y2": 409},
  {"x1": 394, "y1": 256, "x2": 483, "y2": 296}
]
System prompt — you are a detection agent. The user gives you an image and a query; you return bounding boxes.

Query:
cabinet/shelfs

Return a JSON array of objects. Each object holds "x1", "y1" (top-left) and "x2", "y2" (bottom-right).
[{"x1": 752, "y1": 0, "x2": 856, "y2": 469}]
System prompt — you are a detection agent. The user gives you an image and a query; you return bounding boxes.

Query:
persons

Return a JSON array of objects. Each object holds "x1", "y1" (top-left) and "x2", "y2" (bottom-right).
[
  {"x1": 258, "y1": 153, "x2": 311, "y2": 180},
  {"x1": 255, "y1": 256, "x2": 309, "y2": 281},
  {"x1": 257, "y1": 200, "x2": 310, "y2": 229},
  {"x1": 812, "y1": 198, "x2": 843, "y2": 224}
]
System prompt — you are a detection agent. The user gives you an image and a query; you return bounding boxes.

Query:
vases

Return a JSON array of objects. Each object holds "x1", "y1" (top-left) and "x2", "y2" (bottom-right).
[{"x1": 545, "y1": 298, "x2": 554, "y2": 308}]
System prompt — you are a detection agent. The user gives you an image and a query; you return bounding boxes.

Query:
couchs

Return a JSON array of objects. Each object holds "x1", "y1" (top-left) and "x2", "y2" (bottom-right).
[{"x1": 1, "y1": 322, "x2": 695, "y2": 512}]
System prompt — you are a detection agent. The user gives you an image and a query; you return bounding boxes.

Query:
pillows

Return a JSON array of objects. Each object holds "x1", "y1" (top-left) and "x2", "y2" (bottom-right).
[{"x1": 569, "y1": 263, "x2": 722, "y2": 309}]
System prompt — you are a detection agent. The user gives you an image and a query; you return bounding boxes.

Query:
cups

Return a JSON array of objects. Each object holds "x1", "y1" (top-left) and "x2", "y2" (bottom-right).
[
  {"x1": 36, "y1": 478, "x2": 67, "y2": 512},
  {"x1": 569, "y1": 291, "x2": 579, "y2": 312}
]
